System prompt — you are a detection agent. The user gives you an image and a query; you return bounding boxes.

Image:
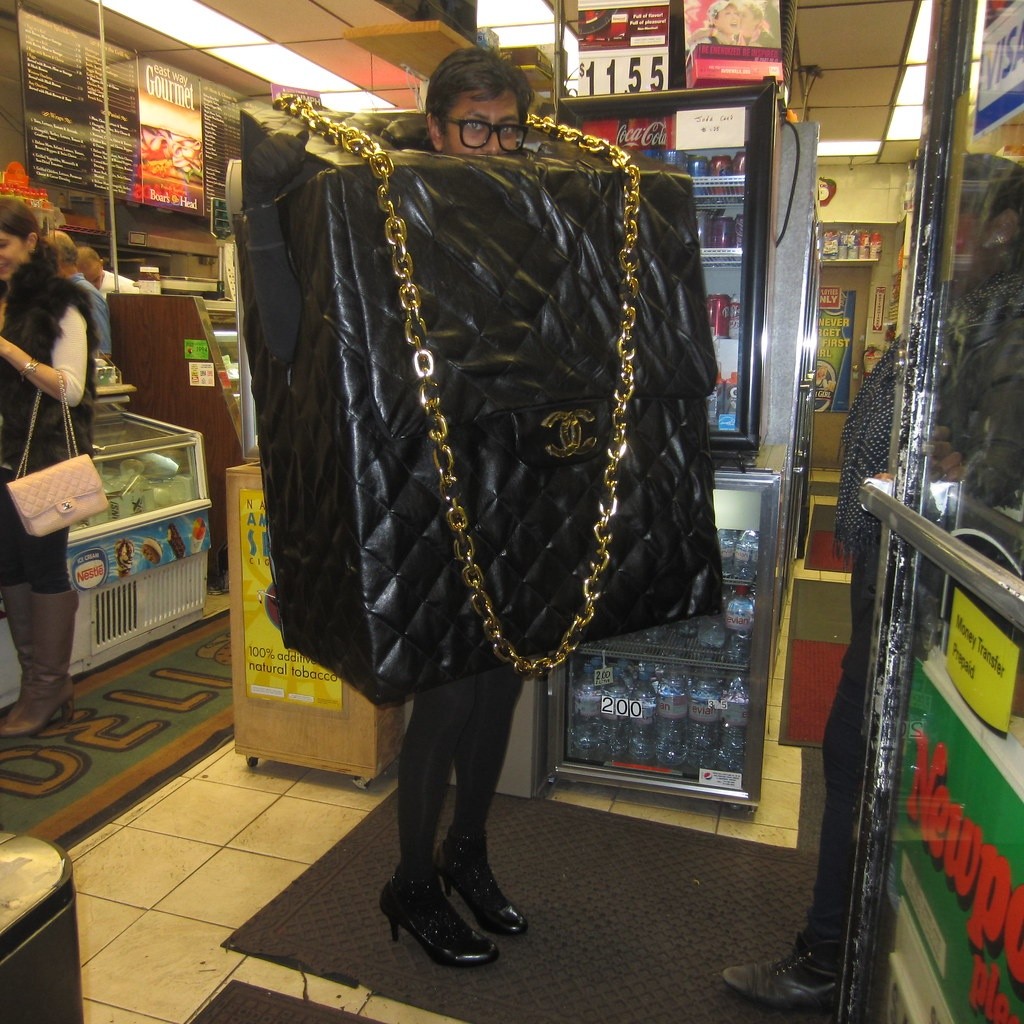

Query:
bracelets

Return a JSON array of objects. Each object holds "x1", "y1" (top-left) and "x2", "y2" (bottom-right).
[{"x1": 19, "y1": 358, "x2": 38, "y2": 383}]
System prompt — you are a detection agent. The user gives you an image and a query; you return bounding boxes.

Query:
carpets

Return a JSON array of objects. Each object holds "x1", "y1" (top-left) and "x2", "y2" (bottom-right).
[{"x1": 0, "y1": 503, "x2": 850, "y2": 1024}]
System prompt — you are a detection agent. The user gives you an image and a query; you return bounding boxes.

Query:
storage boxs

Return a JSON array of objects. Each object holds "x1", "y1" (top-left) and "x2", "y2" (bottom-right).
[
  {"x1": 501, "y1": 46, "x2": 552, "y2": 82},
  {"x1": 685, "y1": 44, "x2": 784, "y2": 88},
  {"x1": 476, "y1": 28, "x2": 500, "y2": 52}
]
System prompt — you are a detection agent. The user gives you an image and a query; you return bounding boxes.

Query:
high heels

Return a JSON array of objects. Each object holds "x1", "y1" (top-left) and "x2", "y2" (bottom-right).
[
  {"x1": 436, "y1": 826, "x2": 527, "y2": 937},
  {"x1": 379, "y1": 864, "x2": 500, "y2": 967}
]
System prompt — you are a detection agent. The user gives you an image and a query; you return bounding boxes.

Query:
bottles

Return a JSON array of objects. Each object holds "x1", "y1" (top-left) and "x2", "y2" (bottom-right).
[{"x1": 560, "y1": 525, "x2": 755, "y2": 780}]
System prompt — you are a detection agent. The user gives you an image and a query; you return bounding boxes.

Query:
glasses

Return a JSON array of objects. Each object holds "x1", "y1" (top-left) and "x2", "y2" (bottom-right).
[{"x1": 440, "y1": 116, "x2": 530, "y2": 156}]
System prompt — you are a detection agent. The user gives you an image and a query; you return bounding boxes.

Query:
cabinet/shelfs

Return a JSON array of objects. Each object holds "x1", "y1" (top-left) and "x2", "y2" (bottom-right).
[{"x1": 226, "y1": 462, "x2": 405, "y2": 786}]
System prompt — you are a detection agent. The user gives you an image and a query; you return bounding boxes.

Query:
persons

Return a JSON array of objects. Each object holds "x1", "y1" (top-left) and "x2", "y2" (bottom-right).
[
  {"x1": 44, "y1": 230, "x2": 113, "y2": 361},
  {"x1": 377, "y1": 47, "x2": 528, "y2": 965},
  {"x1": 715, "y1": 324, "x2": 902, "y2": 1011},
  {"x1": 0, "y1": 195, "x2": 101, "y2": 734},
  {"x1": 76, "y1": 247, "x2": 139, "y2": 304}
]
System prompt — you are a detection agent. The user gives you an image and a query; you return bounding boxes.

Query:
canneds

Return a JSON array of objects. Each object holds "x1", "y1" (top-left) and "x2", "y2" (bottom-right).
[
  {"x1": 706, "y1": 292, "x2": 740, "y2": 340},
  {"x1": 639, "y1": 147, "x2": 746, "y2": 248}
]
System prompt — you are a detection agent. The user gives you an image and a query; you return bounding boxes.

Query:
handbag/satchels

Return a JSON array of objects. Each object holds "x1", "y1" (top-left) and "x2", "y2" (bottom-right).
[
  {"x1": 6, "y1": 367, "x2": 108, "y2": 537},
  {"x1": 230, "y1": 91, "x2": 726, "y2": 707}
]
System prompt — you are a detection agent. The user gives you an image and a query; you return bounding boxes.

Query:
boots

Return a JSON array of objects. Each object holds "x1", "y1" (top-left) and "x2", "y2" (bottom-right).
[{"x1": 0, "y1": 582, "x2": 79, "y2": 736}]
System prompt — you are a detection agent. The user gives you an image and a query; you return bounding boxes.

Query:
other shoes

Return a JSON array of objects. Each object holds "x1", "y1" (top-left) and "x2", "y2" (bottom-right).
[{"x1": 721, "y1": 931, "x2": 840, "y2": 1012}]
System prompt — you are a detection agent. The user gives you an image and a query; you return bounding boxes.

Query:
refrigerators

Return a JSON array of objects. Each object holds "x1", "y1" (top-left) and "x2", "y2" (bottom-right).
[{"x1": 536, "y1": 77, "x2": 802, "y2": 827}]
